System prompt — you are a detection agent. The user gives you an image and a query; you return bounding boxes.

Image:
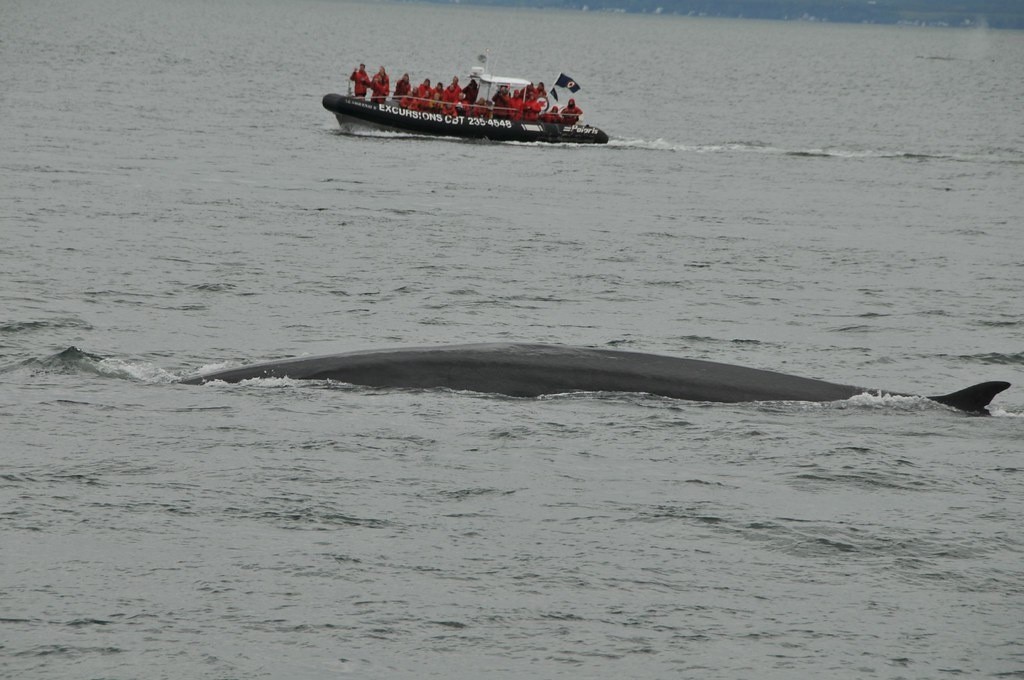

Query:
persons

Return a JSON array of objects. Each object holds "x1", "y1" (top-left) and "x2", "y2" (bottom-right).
[{"x1": 350, "y1": 63, "x2": 583, "y2": 125}]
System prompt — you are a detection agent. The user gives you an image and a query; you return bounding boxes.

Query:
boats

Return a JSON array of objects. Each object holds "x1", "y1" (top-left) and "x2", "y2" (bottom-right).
[{"x1": 320, "y1": 48, "x2": 610, "y2": 145}]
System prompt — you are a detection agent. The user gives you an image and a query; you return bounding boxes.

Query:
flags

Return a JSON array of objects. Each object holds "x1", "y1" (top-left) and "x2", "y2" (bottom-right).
[{"x1": 555, "y1": 73, "x2": 580, "y2": 93}]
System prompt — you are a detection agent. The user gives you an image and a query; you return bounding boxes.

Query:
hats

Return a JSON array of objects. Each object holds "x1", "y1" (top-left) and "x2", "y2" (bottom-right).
[
  {"x1": 553, "y1": 106, "x2": 558, "y2": 111},
  {"x1": 514, "y1": 90, "x2": 520, "y2": 94},
  {"x1": 528, "y1": 91, "x2": 534, "y2": 95},
  {"x1": 569, "y1": 98, "x2": 575, "y2": 102}
]
